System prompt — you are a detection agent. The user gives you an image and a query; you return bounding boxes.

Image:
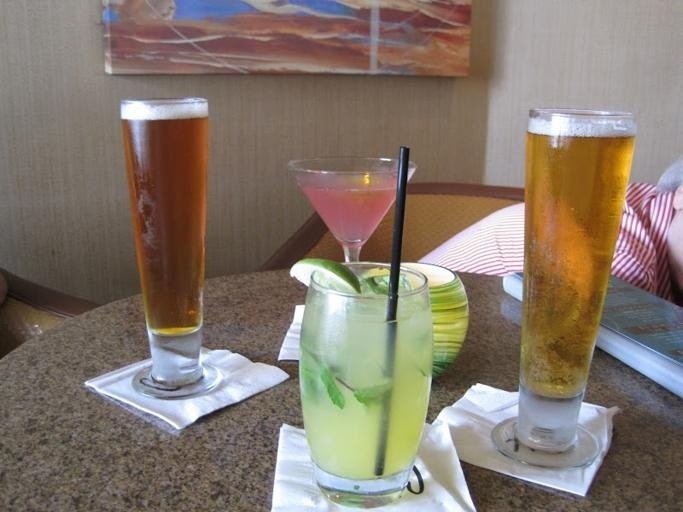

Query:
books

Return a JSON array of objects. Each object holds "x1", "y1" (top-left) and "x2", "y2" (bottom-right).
[{"x1": 503, "y1": 270, "x2": 679, "y2": 399}]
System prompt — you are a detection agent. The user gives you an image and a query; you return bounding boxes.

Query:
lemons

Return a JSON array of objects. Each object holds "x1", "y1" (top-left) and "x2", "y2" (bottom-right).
[{"x1": 289, "y1": 254, "x2": 363, "y2": 295}]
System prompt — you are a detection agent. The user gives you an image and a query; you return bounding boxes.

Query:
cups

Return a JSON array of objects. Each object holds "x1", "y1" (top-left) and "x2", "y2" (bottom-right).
[
  {"x1": 383, "y1": 261, "x2": 469, "y2": 381},
  {"x1": 300, "y1": 268, "x2": 434, "y2": 507},
  {"x1": 118, "y1": 97, "x2": 224, "y2": 401},
  {"x1": 490, "y1": 108, "x2": 638, "y2": 472}
]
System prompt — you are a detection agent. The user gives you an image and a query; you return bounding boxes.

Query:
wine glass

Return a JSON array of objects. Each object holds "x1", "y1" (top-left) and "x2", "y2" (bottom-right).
[{"x1": 287, "y1": 157, "x2": 418, "y2": 264}]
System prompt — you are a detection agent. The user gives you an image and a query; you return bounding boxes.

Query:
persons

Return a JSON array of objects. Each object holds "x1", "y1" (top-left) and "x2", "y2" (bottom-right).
[{"x1": 415, "y1": 182, "x2": 679, "y2": 301}]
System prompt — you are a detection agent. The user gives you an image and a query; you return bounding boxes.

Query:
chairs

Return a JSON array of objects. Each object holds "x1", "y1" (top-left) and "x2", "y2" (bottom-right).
[
  {"x1": 0, "y1": 266, "x2": 101, "y2": 359},
  {"x1": 254, "y1": 182, "x2": 524, "y2": 273}
]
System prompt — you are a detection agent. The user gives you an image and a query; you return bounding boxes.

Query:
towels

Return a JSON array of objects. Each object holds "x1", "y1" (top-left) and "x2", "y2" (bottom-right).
[
  {"x1": 433, "y1": 381, "x2": 622, "y2": 499},
  {"x1": 81, "y1": 343, "x2": 289, "y2": 431},
  {"x1": 275, "y1": 301, "x2": 312, "y2": 362},
  {"x1": 273, "y1": 420, "x2": 475, "y2": 508}
]
